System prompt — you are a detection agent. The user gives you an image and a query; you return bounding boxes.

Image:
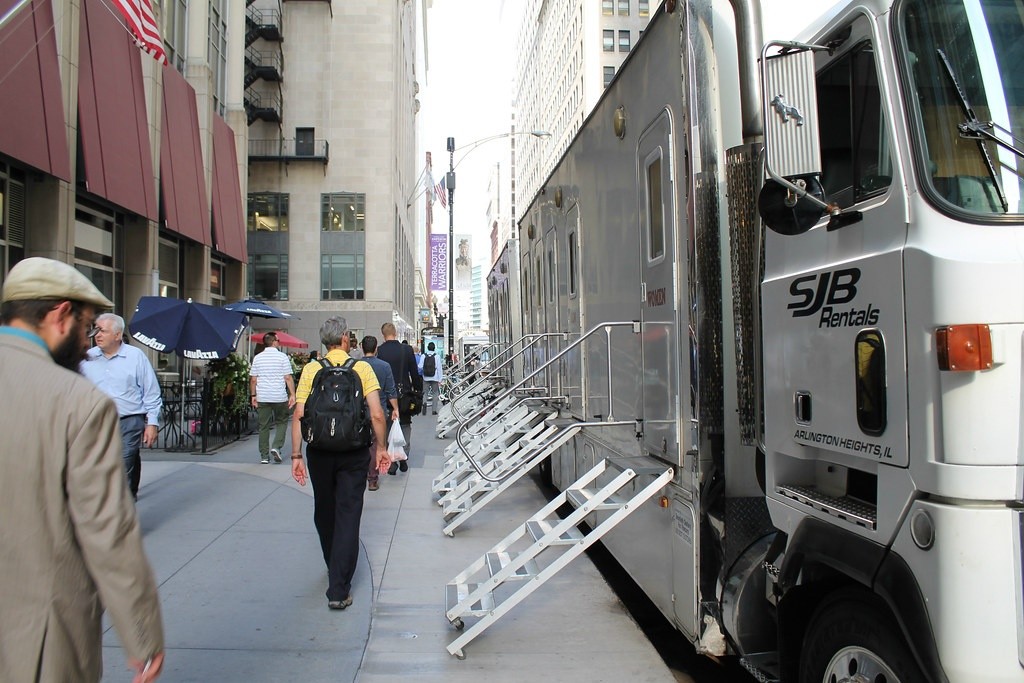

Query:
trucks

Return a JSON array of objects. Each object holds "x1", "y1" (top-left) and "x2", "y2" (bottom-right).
[{"x1": 433, "y1": 0, "x2": 1024, "y2": 683}]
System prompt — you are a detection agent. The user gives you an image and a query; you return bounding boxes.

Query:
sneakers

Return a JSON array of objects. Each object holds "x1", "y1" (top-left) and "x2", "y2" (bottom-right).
[
  {"x1": 271, "y1": 449, "x2": 282, "y2": 462},
  {"x1": 261, "y1": 459, "x2": 271, "y2": 464}
]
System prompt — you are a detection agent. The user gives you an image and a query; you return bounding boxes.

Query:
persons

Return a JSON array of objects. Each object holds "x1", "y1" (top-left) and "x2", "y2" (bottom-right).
[
  {"x1": 250, "y1": 317, "x2": 451, "y2": 608},
  {"x1": 78, "y1": 315, "x2": 163, "y2": 500},
  {"x1": 0, "y1": 255, "x2": 166, "y2": 683}
]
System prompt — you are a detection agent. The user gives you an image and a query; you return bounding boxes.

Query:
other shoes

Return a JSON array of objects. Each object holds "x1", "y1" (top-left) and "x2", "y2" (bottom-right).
[
  {"x1": 328, "y1": 595, "x2": 353, "y2": 609},
  {"x1": 368, "y1": 482, "x2": 378, "y2": 490},
  {"x1": 422, "y1": 405, "x2": 427, "y2": 415},
  {"x1": 400, "y1": 461, "x2": 408, "y2": 471},
  {"x1": 389, "y1": 463, "x2": 398, "y2": 475},
  {"x1": 432, "y1": 411, "x2": 437, "y2": 414}
]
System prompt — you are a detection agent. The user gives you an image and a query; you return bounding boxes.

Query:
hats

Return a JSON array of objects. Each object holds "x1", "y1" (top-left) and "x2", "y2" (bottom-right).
[{"x1": 3, "y1": 257, "x2": 114, "y2": 309}]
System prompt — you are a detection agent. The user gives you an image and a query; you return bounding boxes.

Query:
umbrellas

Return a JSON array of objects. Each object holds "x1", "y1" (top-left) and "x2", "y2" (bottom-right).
[{"x1": 129, "y1": 296, "x2": 309, "y2": 433}]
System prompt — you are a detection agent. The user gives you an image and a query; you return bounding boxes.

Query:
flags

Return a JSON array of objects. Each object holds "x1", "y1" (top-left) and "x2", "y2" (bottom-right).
[
  {"x1": 113, "y1": 0, "x2": 166, "y2": 67},
  {"x1": 434, "y1": 177, "x2": 446, "y2": 208}
]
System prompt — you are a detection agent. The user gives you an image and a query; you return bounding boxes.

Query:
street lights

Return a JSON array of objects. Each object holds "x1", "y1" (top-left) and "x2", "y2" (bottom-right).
[{"x1": 447, "y1": 132, "x2": 552, "y2": 378}]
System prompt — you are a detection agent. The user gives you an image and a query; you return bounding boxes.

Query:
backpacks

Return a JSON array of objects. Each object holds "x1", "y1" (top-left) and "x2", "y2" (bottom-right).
[
  {"x1": 423, "y1": 353, "x2": 437, "y2": 376},
  {"x1": 299, "y1": 358, "x2": 372, "y2": 449}
]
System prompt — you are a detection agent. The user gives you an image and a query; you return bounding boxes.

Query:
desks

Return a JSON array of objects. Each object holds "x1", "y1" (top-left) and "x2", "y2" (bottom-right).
[{"x1": 157, "y1": 385, "x2": 202, "y2": 449}]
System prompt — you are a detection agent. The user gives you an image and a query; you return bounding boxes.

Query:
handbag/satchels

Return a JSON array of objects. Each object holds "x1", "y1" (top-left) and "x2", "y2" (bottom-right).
[
  {"x1": 386, "y1": 418, "x2": 408, "y2": 462},
  {"x1": 400, "y1": 389, "x2": 422, "y2": 415}
]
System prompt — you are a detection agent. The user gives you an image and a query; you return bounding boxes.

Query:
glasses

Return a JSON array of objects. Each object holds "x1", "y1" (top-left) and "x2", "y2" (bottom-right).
[
  {"x1": 275, "y1": 339, "x2": 279, "y2": 343},
  {"x1": 51, "y1": 302, "x2": 101, "y2": 337}
]
